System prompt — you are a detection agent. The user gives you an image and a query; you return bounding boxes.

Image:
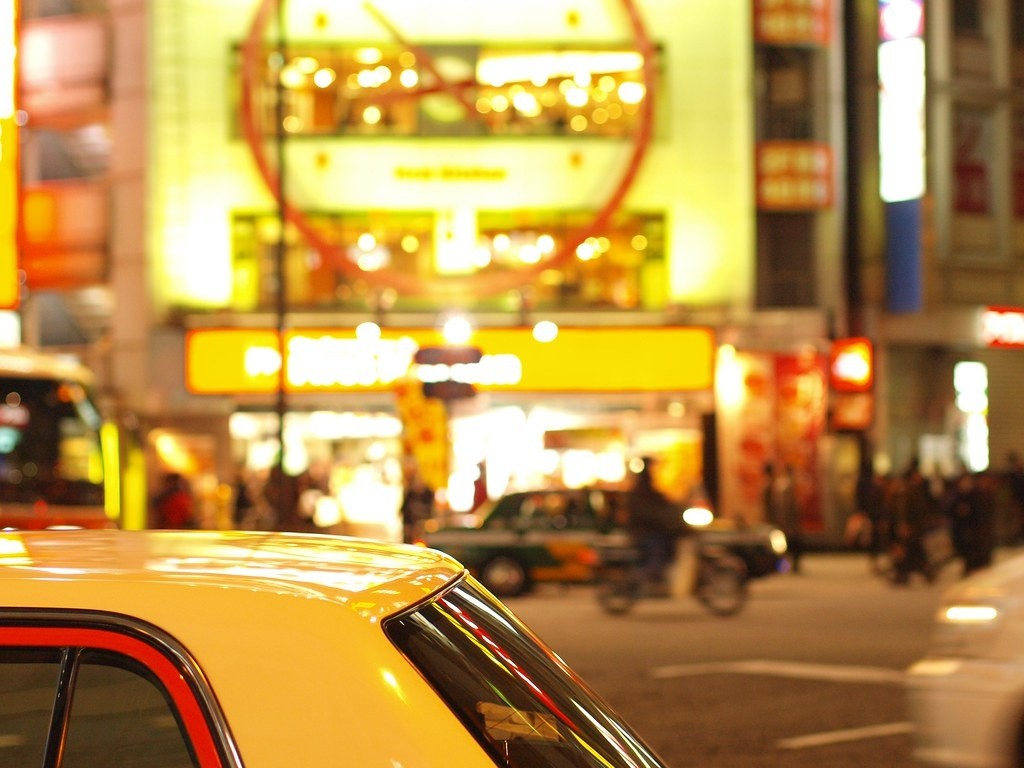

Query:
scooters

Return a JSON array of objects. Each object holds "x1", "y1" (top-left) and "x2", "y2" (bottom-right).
[{"x1": 575, "y1": 501, "x2": 751, "y2": 617}]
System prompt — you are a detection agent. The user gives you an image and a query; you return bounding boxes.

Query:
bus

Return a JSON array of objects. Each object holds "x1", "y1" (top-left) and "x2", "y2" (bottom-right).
[{"x1": 1, "y1": 348, "x2": 120, "y2": 538}]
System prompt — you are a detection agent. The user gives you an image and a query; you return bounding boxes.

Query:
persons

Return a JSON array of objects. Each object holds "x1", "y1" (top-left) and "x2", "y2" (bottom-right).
[
  {"x1": 149, "y1": 449, "x2": 494, "y2": 547},
  {"x1": 626, "y1": 432, "x2": 998, "y2": 602}
]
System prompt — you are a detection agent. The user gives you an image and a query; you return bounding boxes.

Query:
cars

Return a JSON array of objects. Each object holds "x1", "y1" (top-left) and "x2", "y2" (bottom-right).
[
  {"x1": 0, "y1": 528, "x2": 667, "y2": 768},
  {"x1": 413, "y1": 486, "x2": 792, "y2": 603}
]
